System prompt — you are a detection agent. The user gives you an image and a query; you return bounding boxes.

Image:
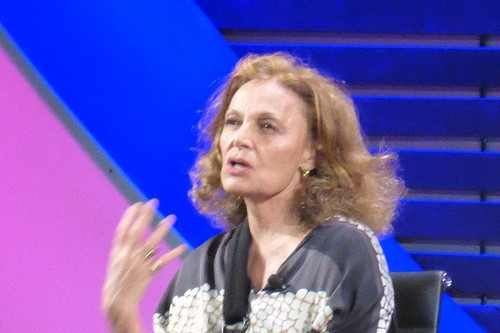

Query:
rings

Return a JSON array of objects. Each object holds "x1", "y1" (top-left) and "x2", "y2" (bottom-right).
[
  {"x1": 139, "y1": 240, "x2": 156, "y2": 261},
  {"x1": 148, "y1": 259, "x2": 163, "y2": 273}
]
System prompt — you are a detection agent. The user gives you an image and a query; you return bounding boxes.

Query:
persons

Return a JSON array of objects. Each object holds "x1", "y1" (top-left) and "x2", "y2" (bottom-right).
[{"x1": 100, "y1": 51, "x2": 408, "y2": 333}]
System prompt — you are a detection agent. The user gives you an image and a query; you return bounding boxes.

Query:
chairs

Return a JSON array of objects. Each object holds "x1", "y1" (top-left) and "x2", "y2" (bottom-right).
[{"x1": 387, "y1": 270, "x2": 453, "y2": 333}]
